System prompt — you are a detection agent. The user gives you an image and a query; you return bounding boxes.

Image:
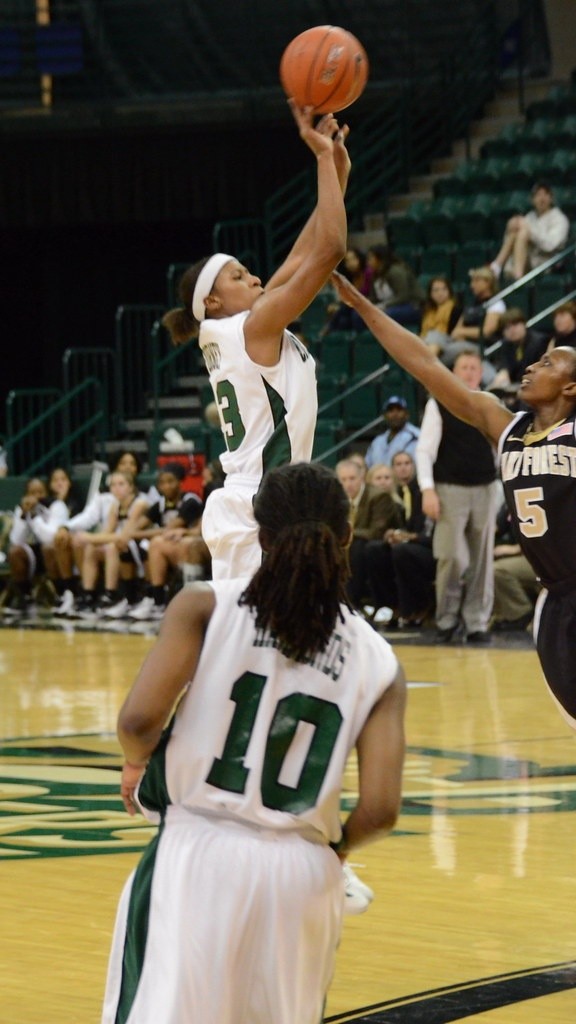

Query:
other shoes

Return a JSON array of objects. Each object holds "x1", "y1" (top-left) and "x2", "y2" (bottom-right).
[
  {"x1": 435, "y1": 622, "x2": 458, "y2": 644},
  {"x1": 467, "y1": 629, "x2": 492, "y2": 644},
  {"x1": 362, "y1": 603, "x2": 376, "y2": 624},
  {"x1": 376, "y1": 607, "x2": 395, "y2": 624},
  {"x1": 341, "y1": 865, "x2": 371, "y2": 910}
]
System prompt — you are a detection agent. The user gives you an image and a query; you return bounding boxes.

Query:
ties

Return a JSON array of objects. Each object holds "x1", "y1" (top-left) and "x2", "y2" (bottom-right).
[{"x1": 402, "y1": 484, "x2": 412, "y2": 522}]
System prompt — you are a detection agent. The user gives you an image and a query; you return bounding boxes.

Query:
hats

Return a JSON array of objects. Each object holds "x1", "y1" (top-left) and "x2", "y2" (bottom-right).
[{"x1": 383, "y1": 396, "x2": 406, "y2": 411}]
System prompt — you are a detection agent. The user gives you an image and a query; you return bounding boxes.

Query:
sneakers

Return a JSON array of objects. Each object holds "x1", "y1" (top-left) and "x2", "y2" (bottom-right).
[
  {"x1": 1, "y1": 585, "x2": 171, "y2": 620},
  {"x1": 4, "y1": 615, "x2": 161, "y2": 635}
]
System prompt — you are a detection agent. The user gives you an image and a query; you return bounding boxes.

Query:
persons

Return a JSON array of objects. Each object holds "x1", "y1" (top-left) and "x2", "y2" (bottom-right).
[
  {"x1": 0, "y1": 402, "x2": 224, "y2": 623},
  {"x1": 335, "y1": 305, "x2": 575, "y2": 630},
  {"x1": 328, "y1": 271, "x2": 576, "y2": 721},
  {"x1": 180, "y1": 93, "x2": 352, "y2": 580},
  {"x1": 414, "y1": 349, "x2": 507, "y2": 642},
  {"x1": 101, "y1": 463, "x2": 406, "y2": 1024},
  {"x1": 313, "y1": 186, "x2": 569, "y2": 372}
]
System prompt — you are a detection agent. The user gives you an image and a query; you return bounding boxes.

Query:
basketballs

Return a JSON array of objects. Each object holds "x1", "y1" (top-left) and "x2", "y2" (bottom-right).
[{"x1": 281, "y1": 24, "x2": 369, "y2": 115}]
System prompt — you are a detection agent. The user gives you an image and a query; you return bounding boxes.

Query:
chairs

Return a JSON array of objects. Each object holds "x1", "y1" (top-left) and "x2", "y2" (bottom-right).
[{"x1": 158, "y1": 79, "x2": 576, "y2": 470}]
